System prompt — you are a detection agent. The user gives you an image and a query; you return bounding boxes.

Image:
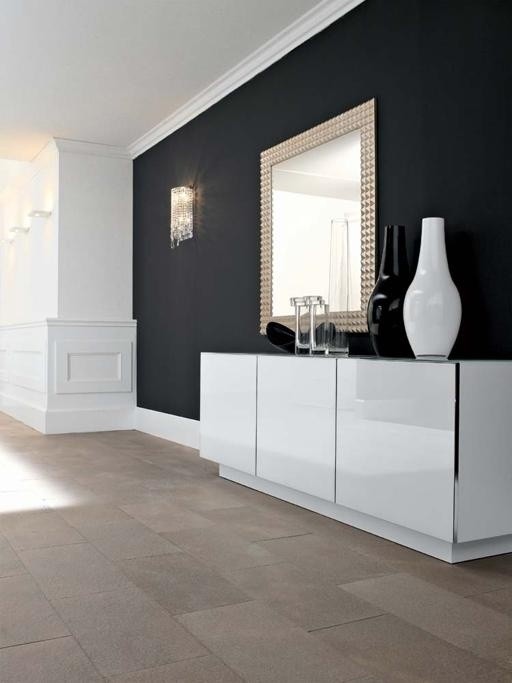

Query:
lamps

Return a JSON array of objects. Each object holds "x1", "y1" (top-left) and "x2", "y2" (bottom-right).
[{"x1": 170, "y1": 186, "x2": 194, "y2": 248}]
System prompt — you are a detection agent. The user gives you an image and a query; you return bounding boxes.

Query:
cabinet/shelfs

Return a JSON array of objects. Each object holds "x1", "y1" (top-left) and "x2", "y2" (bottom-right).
[
  {"x1": 335, "y1": 357, "x2": 457, "y2": 545},
  {"x1": 200, "y1": 352, "x2": 257, "y2": 475},
  {"x1": 257, "y1": 354, "x2": 336, "y2": 501}
]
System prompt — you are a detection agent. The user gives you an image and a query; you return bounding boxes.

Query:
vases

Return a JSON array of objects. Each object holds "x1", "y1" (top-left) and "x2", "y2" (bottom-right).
[
  {"x1": 403, "y1": 217, "x2": 462, "y2": 362},
  {"x1": 366, "y1": 225, "x2": 411, "y2": 358}
]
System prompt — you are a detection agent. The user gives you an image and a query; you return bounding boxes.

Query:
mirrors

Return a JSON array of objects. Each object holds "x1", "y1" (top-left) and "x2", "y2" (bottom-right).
[{"x1": 260, "y1": 98, "x2": 376, "y2": 334}]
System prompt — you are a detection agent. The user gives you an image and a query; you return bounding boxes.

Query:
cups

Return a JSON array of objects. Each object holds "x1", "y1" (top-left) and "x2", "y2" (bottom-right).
[{"x1": 290, "y1": 219, "x2": 350, "y2": 358}]
